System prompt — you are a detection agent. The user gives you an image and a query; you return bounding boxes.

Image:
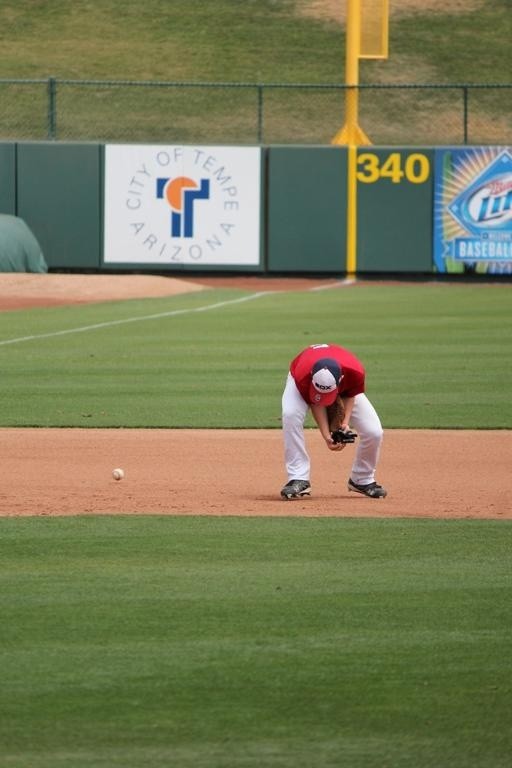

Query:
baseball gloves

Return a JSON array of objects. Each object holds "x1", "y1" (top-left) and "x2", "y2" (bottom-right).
[{"x1": 331, "y1": 427, "x2": 358, "y2": 443}]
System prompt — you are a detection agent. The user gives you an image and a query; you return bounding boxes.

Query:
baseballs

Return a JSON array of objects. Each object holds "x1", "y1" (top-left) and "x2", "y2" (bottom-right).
[{"x1": 113, "y1": 468, "x2": 124, "y2": 480}]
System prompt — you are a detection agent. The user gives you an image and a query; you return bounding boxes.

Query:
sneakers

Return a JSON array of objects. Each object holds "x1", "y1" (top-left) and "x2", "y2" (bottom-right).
[
  {"x1": 347, "y1": 478, "x2": 387, "y2": 498},
  {"x1": 281, "y1": 480, "x2": 311, "y2": 499}
]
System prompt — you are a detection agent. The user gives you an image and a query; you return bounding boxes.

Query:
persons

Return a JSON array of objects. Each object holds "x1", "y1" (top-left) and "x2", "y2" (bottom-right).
[{"x1": 280, "y1": 342, "x2": 389, "y2": 500}]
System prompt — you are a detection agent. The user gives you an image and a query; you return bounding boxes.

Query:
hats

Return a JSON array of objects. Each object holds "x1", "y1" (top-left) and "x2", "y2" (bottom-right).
[{"x1": 309, "y1": 358, "x2": 340, "y2": 406}]
